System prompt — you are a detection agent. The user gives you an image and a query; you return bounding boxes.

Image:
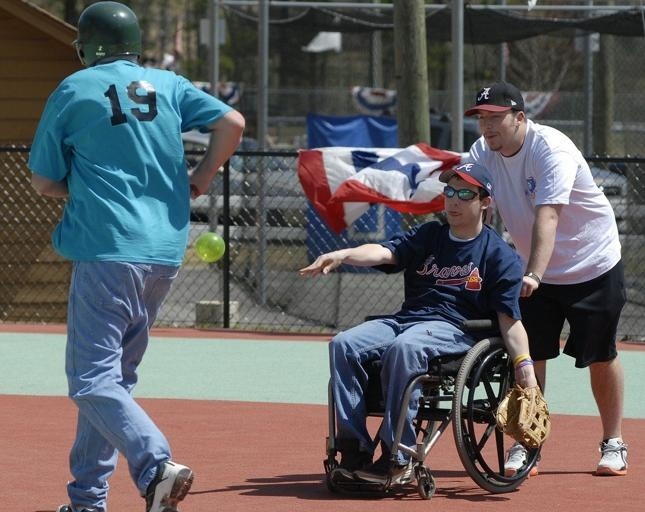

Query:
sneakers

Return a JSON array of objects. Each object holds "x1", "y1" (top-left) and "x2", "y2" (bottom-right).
[
  {"x1": 141, "y1": 457, "x2": 194, "y2": 512},
  {"x1": 500, "y1": 434, "x2": 542, "y2": 479},
  {"x1": 330, "y1": 458, "x2": 373, "y2": 486},
  {"x1": 56, "y1": 504, "x2": 73, "y2": 512},
  {"x1": 594, "y1": 434, "x2": 631, "y2": 477},
  {"x1": 352, "y1": 454, "x2": 415, "y2": 486}
]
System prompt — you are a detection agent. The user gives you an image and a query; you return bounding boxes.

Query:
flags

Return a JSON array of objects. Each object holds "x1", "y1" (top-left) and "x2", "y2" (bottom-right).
[
  {"x1": 296, "y1": 140, "x2": 470, "y2": 234},
  {"x1": 305, "y1": 108, "x2": 454, "y2": 275}
]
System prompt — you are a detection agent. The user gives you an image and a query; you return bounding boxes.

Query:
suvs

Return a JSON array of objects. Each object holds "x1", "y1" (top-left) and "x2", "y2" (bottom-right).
[{"x1": 423, "y1": 106, "x2": 631, "y2": 228}]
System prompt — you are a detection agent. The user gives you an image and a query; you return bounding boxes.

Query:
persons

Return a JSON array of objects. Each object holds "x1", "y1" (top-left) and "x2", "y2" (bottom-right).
[
  {"x1": 26, "y1": 0, "x2": 247, "y2": 511},
  {"x1": 467, "y1": 80, "x2": 633, "y2": 478},
  {"x1": 297, "y1": 160, "x2": 539, "y2": 488}
]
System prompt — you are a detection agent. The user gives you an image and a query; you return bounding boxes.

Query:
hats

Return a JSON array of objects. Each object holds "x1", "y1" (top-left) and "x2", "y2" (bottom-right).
[
  {"x1": 436, "y1": 163, "x2": 496, "y2": 197},
  {"x1": 461, "y1": 81, "x2": 526, "y2": 119}
]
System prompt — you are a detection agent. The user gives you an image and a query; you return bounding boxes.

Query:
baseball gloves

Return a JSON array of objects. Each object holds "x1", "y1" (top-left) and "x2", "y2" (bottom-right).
[{"x1": 496, "y1": 384, "x2": 552, "y2": 449}]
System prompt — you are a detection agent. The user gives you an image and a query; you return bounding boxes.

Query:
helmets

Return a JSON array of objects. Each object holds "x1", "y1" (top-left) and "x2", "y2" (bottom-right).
[{"x1": 76, "y1": 0, "x2": 143, "y2": 68}]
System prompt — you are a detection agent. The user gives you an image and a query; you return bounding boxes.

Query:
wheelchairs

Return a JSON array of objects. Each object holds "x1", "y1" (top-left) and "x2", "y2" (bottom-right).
[{"x1": 320, "y1": 312, "x2": 550, "y2": 499}]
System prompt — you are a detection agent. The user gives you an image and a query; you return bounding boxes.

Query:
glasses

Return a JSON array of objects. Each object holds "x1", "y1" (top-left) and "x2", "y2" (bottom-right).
[{"x1": 443, "y1": 184, "x2": 491, "y2": 200}]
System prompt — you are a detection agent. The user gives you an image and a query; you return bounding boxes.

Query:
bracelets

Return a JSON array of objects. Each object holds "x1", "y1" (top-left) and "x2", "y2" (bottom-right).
[
  {"x1": 524, "y1": 270, "x2": 542, "y2": 286},
  {"x1": 512, "y1": 354, "x2": 537, "y2": 372}
]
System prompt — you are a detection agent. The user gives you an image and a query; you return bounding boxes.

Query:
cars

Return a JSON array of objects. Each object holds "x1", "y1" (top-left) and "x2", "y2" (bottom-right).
[{"x1": 179, "y1": 128, "x2": 310, "y2": 230}]
